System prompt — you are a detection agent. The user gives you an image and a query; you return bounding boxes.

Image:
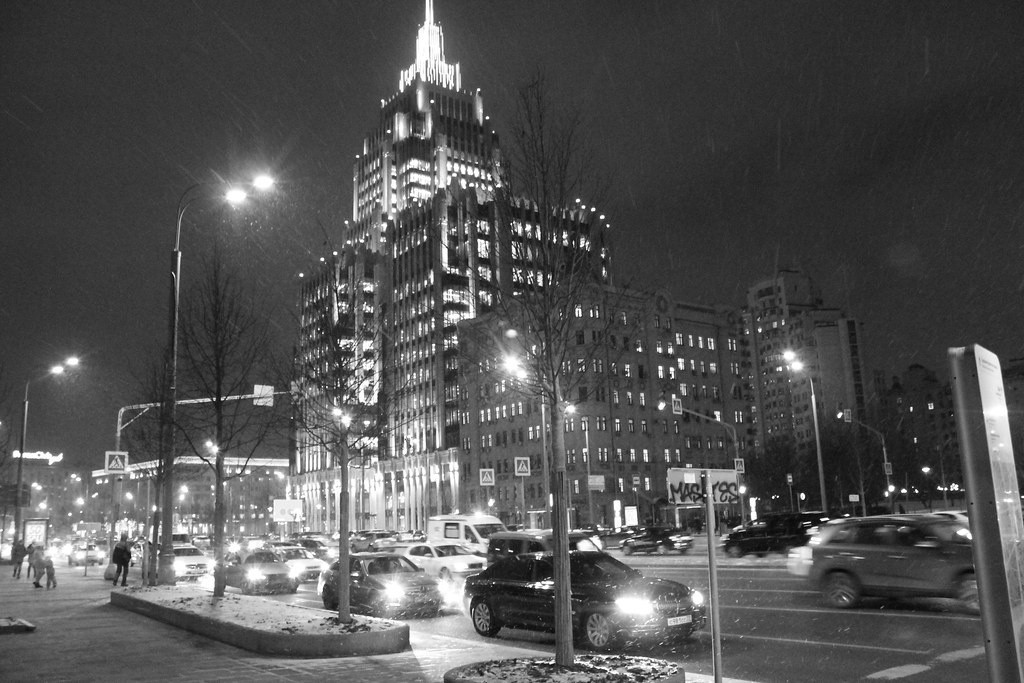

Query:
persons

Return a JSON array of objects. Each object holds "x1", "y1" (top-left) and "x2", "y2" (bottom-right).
[
  {"x1": 113, "y1": 534, "x2": 139, "y2": 587},
  {"x1": 33, "y1": 543, "x2": 47, "y2": 588},
  {"x1": 44, "y1": 556, "x2": 57, "y2": 590},
  {"x1": 27, "y1": 539, "x2": 36, "y2": 578},
  {"x1": 12, "y1": 540, "x2": 26, "y2": 579}
]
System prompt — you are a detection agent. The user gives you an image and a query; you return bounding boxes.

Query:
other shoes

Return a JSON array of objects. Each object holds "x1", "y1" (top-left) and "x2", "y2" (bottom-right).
[
  {"x1": 113, "y1": 580, "x2": 117, "y2": 585},
  {"x1": 121, "y1": 583, "x2": 128, "y2": 586},
  {"x1": 33, "y1": 582, "x2": 43, "y2": 588}
]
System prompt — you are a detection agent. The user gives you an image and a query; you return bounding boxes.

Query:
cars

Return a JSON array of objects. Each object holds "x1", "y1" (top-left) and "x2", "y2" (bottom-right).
[
  {"x1": 465, "y1": 552, "x2": 706, "y2": 652},
  {"x1": 45, "y1": 529, "x2": 693, "y2": 597}
]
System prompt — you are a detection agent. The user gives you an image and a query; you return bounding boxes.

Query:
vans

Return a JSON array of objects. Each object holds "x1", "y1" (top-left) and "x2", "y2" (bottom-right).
[{"x1": 427, "y1": 516, "x2": 507, "y2": 553}]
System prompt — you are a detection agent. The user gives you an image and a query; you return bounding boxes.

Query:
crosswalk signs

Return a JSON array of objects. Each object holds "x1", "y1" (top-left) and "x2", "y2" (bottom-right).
[
  {"x1": 735, "y1": 458, "x2": 744, "y2": 473},
  {"x1": 515, "y1": 457, "x2": 530, "y2": 476},
  {"x1": 479, "y1": 468, "x2": 495, "y2": 486},
  {"x1": 106, "y1": 451, "x2": 128, "y2": 475}
]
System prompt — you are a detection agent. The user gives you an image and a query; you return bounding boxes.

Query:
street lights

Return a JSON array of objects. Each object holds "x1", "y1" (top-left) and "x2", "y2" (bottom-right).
[
  {"x1": 783, "y1": 349, "x2": 826, "y2": 511},
  {"x1": 658, "y1": 401, "x2": 741, "y2": 518},
  {"x1": 511, "y1": 353, "x2": 552, "y2": 530},
  {"x1": 15, "y1": 356, "x2": 80, "y2": 545},
  {"x1": 836, "y1": 410, "x2": 893, "y2": 512},
  {"x1": 159, "y1": 175, "x2": 276, "y2": 586}
]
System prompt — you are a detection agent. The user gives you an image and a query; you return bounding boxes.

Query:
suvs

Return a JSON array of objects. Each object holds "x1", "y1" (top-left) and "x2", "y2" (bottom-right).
[
  {"x1": 720, "y1": 512, "x2": 835, "y2": 559},
  {"x1": 812, "y1": 513, "x2": 981, "y2": 618}
]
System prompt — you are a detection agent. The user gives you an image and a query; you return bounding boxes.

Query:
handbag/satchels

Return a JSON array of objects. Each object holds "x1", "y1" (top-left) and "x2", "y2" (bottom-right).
[{"x1": 119, "y1": 552, "x2": 130, "y2": 565}]
